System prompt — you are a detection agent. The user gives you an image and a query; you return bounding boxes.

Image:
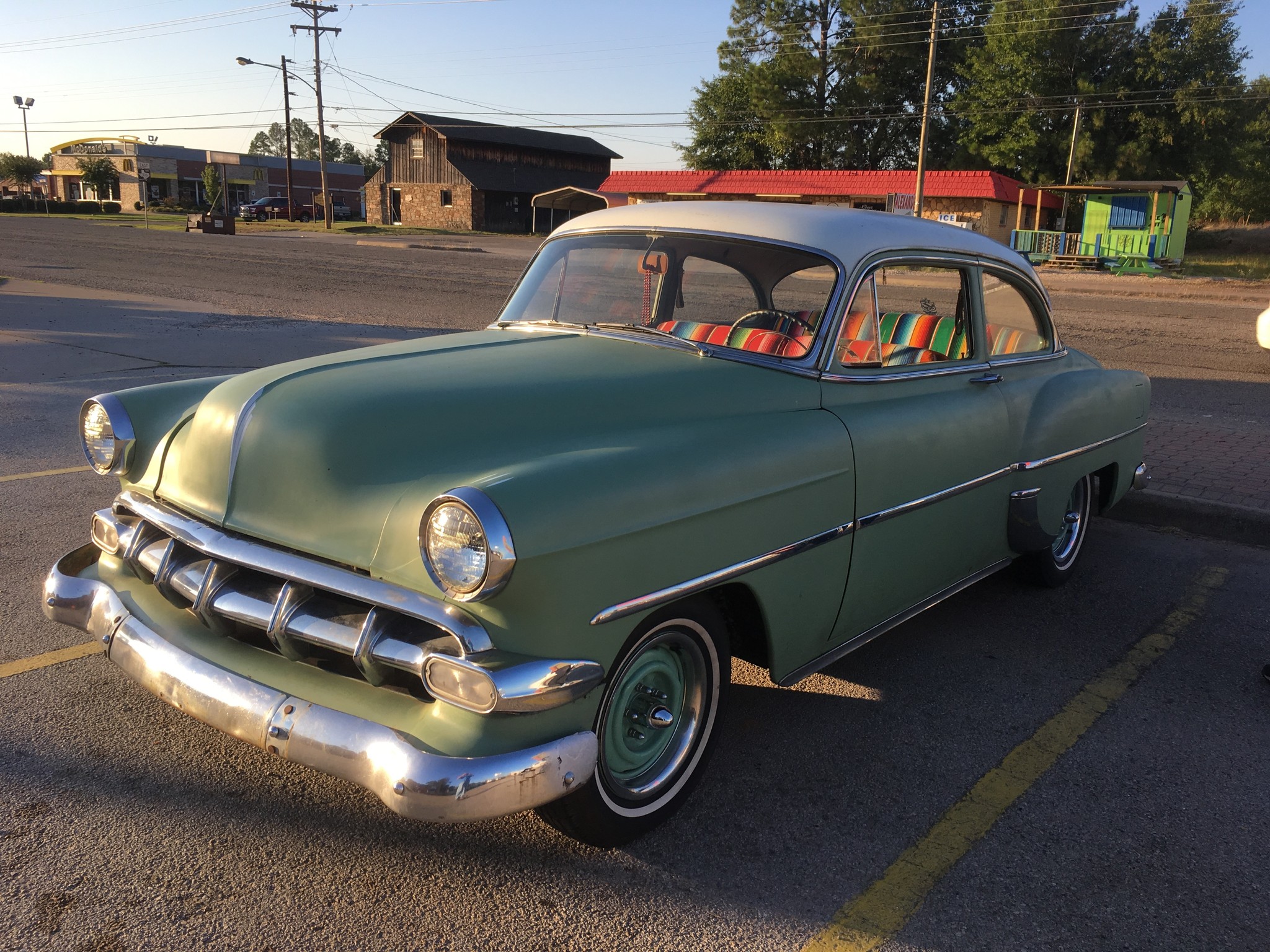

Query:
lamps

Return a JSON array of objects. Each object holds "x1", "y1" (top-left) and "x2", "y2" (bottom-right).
[
  {"x1": 1179, "y1": 193, "x2": 1183, "y2": 201},
  {"x1": 1098, "y1": 196, "x2": 1102, "y2": 199}
]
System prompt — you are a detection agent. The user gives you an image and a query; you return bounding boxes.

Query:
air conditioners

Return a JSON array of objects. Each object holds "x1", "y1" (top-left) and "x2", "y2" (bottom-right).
[{"x1": 945, "y1": 221, "x2": 973, "y2": 230}]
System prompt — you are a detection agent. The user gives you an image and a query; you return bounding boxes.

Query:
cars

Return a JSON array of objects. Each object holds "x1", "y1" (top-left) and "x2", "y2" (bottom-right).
[{"x1": 39, "y1": 197, "x2": 1155, "y2": 844}]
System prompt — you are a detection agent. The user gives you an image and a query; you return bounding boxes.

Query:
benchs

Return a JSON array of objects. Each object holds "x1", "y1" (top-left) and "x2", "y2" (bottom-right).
[
  {"x1": 768, "y1": 307, "x2": 1043, "y2": 356},
  {"x1": 1044, "y1": 260, "x2": 1099, "y2": 269},
  {"x1": 1104, "y1": 262, "x2": 1162, "y2": 272}
]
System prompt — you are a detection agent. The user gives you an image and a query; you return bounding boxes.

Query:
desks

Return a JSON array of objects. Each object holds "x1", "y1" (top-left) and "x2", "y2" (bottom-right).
[
  {"x1": 1111, "y1": 251, "x2": 1154, "y2": 278},
  {"x1": 1015, "y1": 249, "x2": 1041, "y2": 267},
  {"x1": 1052, "y1": 253, "x2": 1101, "y2": 271}
]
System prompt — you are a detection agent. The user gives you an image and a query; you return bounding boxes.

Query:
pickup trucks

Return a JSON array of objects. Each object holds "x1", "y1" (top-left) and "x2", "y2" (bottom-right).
[
  {"x1": 239, "y1": 197, "x2": 318, "y2": 223},
  {"x1": 333, "y1": 200, "x2": 351, "y2": 222}
]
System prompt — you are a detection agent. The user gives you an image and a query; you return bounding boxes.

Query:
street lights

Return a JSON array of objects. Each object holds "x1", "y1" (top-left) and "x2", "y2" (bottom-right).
[
  {"x1": 13, "y1": 95, "x2": 35, "y2": 203},
  {"x1": 235, "y1": 56, "x2": 332, "y2": 230}
]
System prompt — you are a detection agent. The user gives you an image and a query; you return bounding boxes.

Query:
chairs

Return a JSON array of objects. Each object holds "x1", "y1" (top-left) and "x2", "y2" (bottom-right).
[
  {"x1": 656, "y1": 319, "x2": 782, "y2": 358},
  {"x1": 779, "y1": 335, "x2": 938, "y2": 369}
]
